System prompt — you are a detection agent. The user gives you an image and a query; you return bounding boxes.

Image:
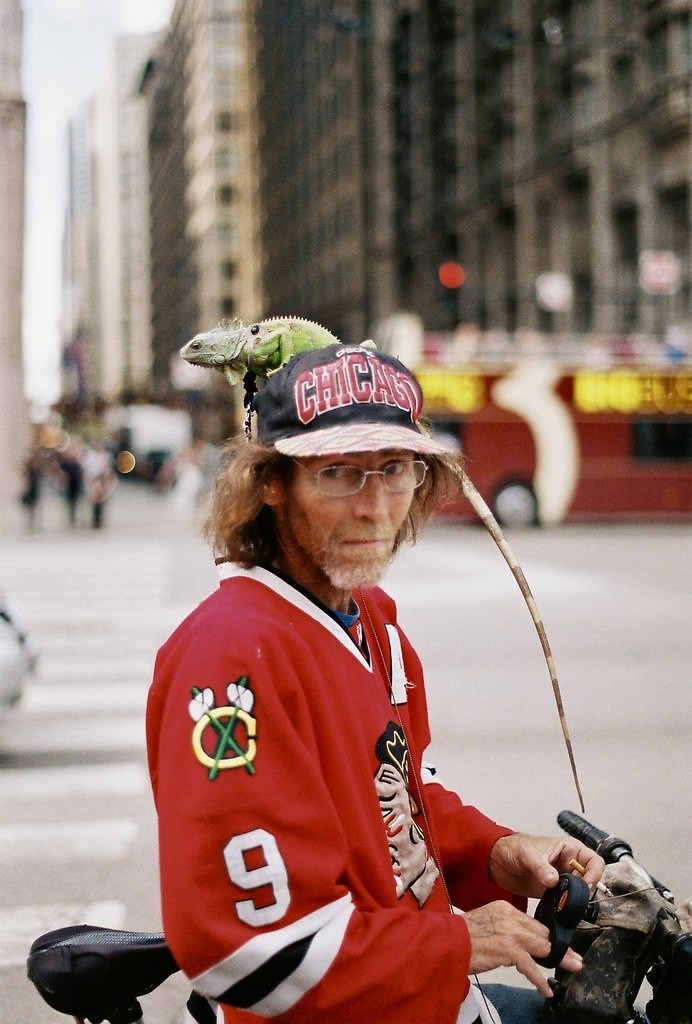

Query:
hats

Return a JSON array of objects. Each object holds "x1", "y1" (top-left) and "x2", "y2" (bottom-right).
[{"x1": 250, "y1": 348, "x2": 455, "y2": 454}]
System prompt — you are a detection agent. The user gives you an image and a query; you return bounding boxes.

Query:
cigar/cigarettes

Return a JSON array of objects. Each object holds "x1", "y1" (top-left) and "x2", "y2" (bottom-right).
[{"x1": 569, "y1": 859, "x2": 613, "y2": 897}]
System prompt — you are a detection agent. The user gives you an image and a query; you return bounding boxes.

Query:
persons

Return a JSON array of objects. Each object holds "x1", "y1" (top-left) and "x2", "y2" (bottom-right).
[
  {"x1": 23, "y1": 441, "x2": 112, "y2": 530},
  {"x1": 145, "y1": 342, "x2": 605, "y2": 1024}
]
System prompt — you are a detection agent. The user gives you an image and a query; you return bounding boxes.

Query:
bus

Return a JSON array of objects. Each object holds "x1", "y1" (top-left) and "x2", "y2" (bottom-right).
[{"x1": 399, "y1": 334, "x2": 692, "y2": 530}]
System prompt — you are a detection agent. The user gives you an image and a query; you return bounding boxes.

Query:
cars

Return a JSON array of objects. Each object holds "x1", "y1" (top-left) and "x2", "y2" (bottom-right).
[{"x1": 0, "y1": 587, "x2": 42, "y2": 708}]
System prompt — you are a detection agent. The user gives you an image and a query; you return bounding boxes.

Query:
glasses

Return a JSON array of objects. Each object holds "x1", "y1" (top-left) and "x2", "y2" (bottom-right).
[{"x1": 289, "y1": 455, "x2": 430, "y2": 494}]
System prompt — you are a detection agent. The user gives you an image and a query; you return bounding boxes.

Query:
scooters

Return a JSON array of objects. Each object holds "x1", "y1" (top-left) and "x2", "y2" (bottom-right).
[{"x1": 27, "y1": 807, "x2": 692, "y2": 1023}]
